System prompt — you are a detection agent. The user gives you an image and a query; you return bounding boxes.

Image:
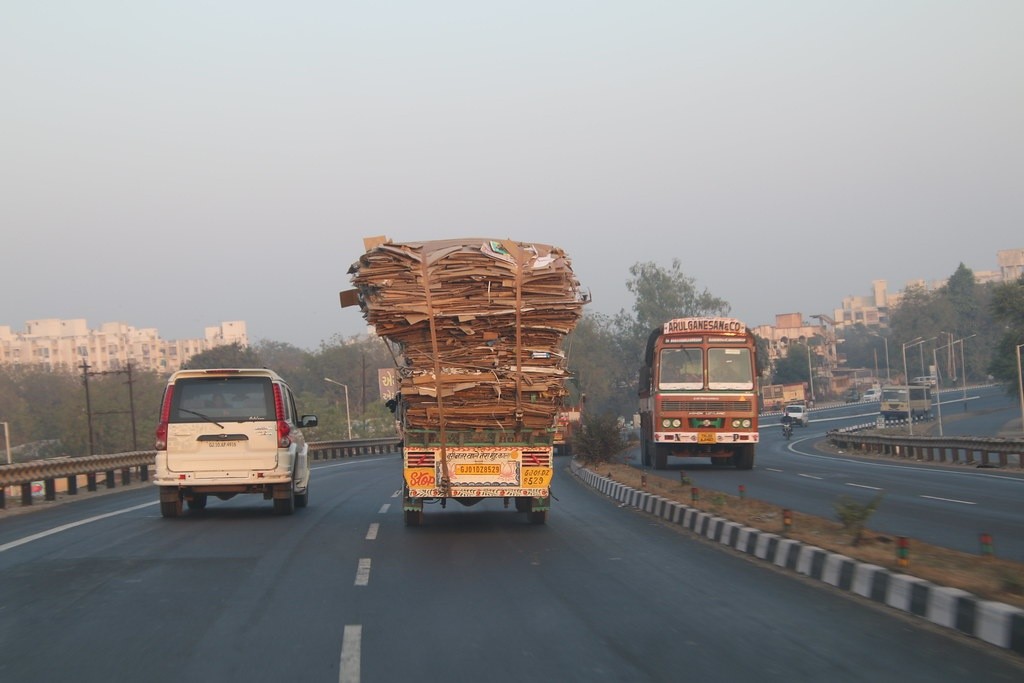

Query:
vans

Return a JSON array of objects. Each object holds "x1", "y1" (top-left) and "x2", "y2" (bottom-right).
[
  {"x1": 784, "y1": 405, "x2": 809, "y2": 427},
  {"x1": 863, "y1": 388, "x2": 882, "y2": 403},
  {"x1": 911, "y1": 376, "x2": 937, "y2": 386}
]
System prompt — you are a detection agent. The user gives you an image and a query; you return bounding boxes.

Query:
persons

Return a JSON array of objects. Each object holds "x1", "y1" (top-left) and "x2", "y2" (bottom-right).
[
  {"x1": 781, "y1": 412, "x2": 793, "y2": 436},
  {"x1": 666, "y1": 364, "x2": 682, "y2": 379}
]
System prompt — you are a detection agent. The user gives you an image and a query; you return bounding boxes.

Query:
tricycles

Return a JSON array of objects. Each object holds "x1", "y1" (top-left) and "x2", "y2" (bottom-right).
[{"x1": 844, "y1": 388, "x2": 862, "y2": 404}]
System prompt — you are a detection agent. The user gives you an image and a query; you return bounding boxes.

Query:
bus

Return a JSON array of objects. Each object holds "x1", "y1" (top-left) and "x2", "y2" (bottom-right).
[{"x1": 880, "y1": 386, "x2": 932, "y2": 420}]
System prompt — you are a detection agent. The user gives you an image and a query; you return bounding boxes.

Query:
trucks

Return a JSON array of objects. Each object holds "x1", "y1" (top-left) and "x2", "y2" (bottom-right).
[
  {"x1": 553, "y1": 378, "x2": 587, "y2": 456},
  {"x1": 638, "y1": 317, "x2": 759, "y2": 471},
  {"x1": 762, "y1": 382, "x2": 810, "y2": 411},
  {"x1": 386, "y1": 379, "x2": 554, "y2": 526}
]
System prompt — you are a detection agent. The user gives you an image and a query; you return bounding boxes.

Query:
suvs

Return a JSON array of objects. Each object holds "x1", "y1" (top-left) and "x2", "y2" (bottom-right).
[{"x1": 154, "y1": 367, "x2": 317, "y2": 517}]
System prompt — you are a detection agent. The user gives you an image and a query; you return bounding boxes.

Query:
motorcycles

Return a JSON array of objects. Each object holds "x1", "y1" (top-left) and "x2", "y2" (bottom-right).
[{"x1": 782, "y1": 422, "x2": 794, "y2": 439}]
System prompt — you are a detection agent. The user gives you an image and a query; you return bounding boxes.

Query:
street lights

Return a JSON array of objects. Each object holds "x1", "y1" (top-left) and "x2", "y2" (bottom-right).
[
  {"x1": 869, "y1": 332, "x2": 890, "y2": 386},
  {"x1": 961, "y1": 334, "x2": 977, "y2": 402},
  {"x1": 324, "y1": 377, "x2": 352, "y2": 440},
  {"x1": 903, "y1": 336, "x2": 923, "y2": 435},
  {"x1": 919, "y1": 337, "x2": 938, "y2": 411},
  {"x1": 797, "y1": 342, "x2": 814, "y2": 400},
  {"x1": 940, "y1": 331, "x2": 950, "y2": 376}
]
